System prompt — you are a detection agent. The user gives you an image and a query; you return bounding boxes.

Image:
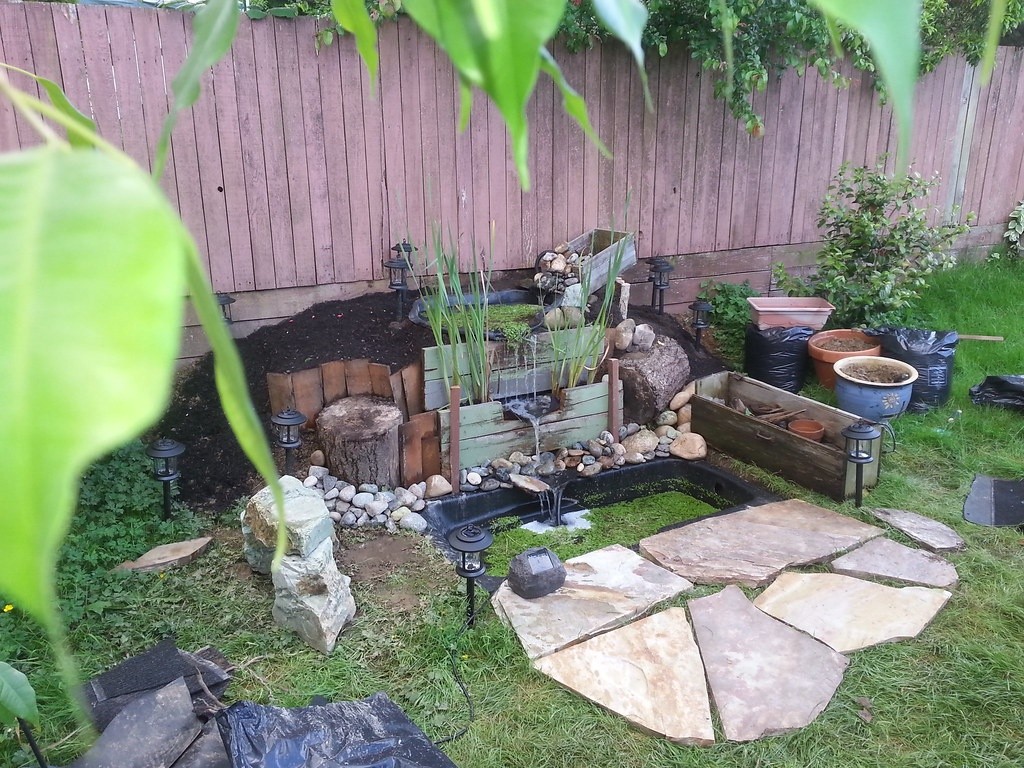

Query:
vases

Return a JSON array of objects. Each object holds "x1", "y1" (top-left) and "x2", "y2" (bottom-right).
[
  {"x1": 746, "y1": 295, "x2": 836, "y2": 331},
  {"x1": 831, "y1": 357, "x2": 920, "y2": 423},
  {"x1": 789, "y1": 420, "x2": 825, "y2": 442},
  {"x1": 808, "y1": 329, "x2": 880, "y2": 389}
]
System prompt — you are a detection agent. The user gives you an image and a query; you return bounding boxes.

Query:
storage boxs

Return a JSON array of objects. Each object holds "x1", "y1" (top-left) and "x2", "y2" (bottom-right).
[
  {"x1": 556, "y1": 229, "x2": 637, "y2": 293},
  {"x1": 688, "y1": 369, "x2": 881, "y2": 502}
]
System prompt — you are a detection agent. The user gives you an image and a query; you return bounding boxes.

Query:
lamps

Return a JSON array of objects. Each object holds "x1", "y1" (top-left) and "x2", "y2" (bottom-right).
[
  {"x1": 688, "y1": 297, "x2": 713, "y2": 330},
  {"x1": 446, "y1": 524, "x2": 494, "y2": 579},
  {"x1": 271, "y1": 406, "x2": 306, "y2": 450},
  {"x1": 215, "y1": 291, "x2": 236, "y2": 326},
  {"x1": 144, "y1": 437, "x2": 184, "y2": 483},
  {"x1": 841, "y1": 420, "x2": 881, "y2": 465},
  {"x1": 383, "y1": 239, "x2": 418, "y2": 291},
  {"x1": 645, "y1": 258, "x2": 671, "y2": 291}
]
